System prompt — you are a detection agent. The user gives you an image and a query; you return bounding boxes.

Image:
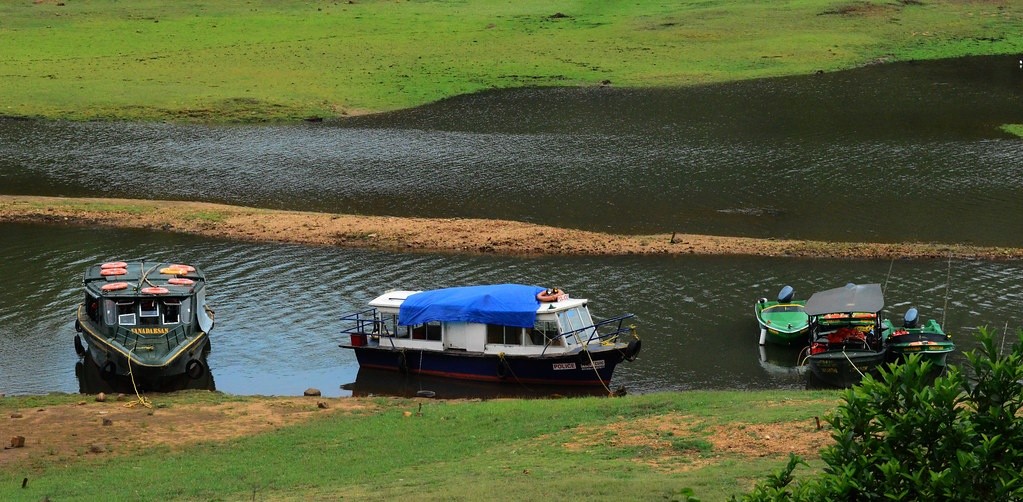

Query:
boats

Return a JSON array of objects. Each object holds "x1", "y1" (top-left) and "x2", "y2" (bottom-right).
[
  {"x1": 754, "y1": 248, "x2": 956, "y2": 386},
  {"x1": 338, "y1": 284, "x2": 642, "y2": 387},
  {"x1": 77, "y1": 260, "x2": 215, "y2": 392}
]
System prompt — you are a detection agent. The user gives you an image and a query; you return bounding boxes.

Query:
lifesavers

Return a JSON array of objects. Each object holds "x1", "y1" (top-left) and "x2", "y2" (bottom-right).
[
  {"x1": 909, "y1": 340, "x2": 937, "y2": 346},
  {"x1": 101, "y1": 261, "x2": 127, "y2": 268},
  {"x1": 73, "y1": 334, "x2": 82, "y2": 355},
  {"x1": 168, "y1": 278, "x2": 193, "y2": 285},
  {"x1": 824, "y1": 312, "x2": 878, "y2": 319},
  {"x1": 625, "y1": 338, "x2": 641, "y2": 362},
  {"x1": 75, "y1": 361, "x2": 83, "y2": 377},
  {"x1": 75, "y1": 319, "x2": 83, "y2": 333},
  {"x1": 168, "y1": 264, "x2": 195, "y2": 272},
  {"x1": 102, "y1": 283, "x2": 127, "y2": 290},
  {"x1": 185, "y1": 359, "x2": 205, "y2": 380},
  {"x1": 98, "y1": 360, "x2": 117, "y2": 382},
  {"x1": 141, "y1": 287, "x2": 168, "y2": 294},
  {"x1": 159, "y1": 267, "x2": 187, "y2": 275},
  {"x1": 537, "y1": 289, "x2": 564, "y2": 301},
  {"x1": 100, "y1": 269, "x2": 127, "y2": 275}
]
